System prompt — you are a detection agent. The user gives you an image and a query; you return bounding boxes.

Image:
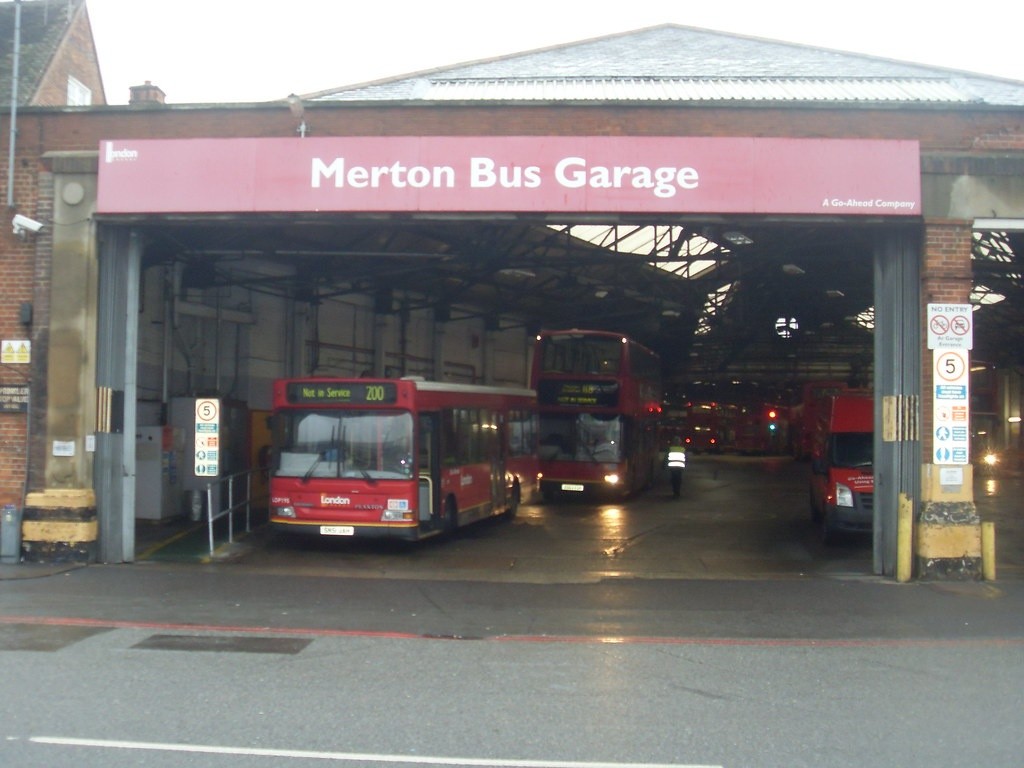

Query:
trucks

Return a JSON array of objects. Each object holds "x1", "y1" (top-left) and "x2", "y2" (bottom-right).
[{"x1": 811, "y1": 390, "x2": 876, "y2": 546}]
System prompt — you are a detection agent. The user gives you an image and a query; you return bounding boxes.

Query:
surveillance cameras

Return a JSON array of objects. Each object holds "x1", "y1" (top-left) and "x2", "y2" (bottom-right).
[{"x1": 11, "y1": 214, "x2": 44, "y2": 233}]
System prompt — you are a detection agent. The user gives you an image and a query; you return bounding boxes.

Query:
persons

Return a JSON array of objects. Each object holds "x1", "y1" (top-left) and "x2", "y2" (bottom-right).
[{"x1": 663, "y1": 436, "x2": 688, "y2": 500}]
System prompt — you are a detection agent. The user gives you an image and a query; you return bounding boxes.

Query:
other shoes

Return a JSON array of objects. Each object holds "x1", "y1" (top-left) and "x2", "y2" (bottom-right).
[{"x1": 672, "y1": 492, "x2": 680, "y2": 500}]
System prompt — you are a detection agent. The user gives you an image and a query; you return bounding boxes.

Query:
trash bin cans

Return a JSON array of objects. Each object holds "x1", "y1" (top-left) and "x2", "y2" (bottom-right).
[{"x1": 186, "y1": 489, "x2": 209, "y2": 522}]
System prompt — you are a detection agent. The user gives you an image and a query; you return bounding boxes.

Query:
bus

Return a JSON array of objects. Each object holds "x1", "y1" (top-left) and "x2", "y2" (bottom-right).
[
  {"x1": 269, "y1": 375, "x2": 541, "y2": 543},
  {"x1": 663, "y1": 378, "x2": 848, "y2": 464},
  {"x1": 528, "y1": 329, "x2": 662, "y2": 502}
]
